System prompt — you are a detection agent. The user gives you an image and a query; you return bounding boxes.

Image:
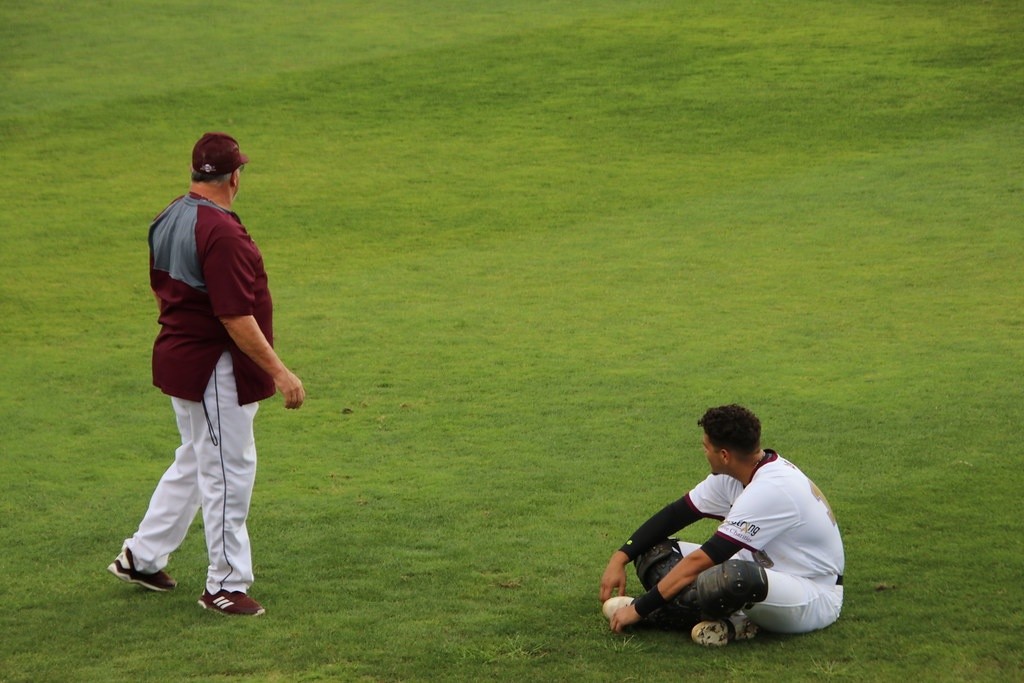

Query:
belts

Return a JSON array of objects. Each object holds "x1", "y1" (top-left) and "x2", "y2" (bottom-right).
[{"x1": 836, "y1": 575, "x2": 843, "y2": 585}]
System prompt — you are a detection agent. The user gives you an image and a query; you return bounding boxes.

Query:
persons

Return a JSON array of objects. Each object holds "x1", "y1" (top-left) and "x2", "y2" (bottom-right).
[
  {"x1": 599, "y1": 403, "x2": 846, "y2": 648},
  {"x1": 103, "y1": 132, "x2": 305, "y2": 616}
]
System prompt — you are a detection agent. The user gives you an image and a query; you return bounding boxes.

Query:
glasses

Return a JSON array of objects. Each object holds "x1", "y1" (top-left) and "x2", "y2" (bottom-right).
[{"x1": 239, "y1": 165, "x2": 245, "y2": 172}]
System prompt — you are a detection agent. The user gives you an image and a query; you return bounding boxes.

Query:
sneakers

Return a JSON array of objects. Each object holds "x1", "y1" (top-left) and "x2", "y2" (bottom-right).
[
  {"x1": 107, "y1": 542, "x2": 176, "y2": 591},
  {"x1": 197, "y1": 588, "x2": 265, "y2": 617}
]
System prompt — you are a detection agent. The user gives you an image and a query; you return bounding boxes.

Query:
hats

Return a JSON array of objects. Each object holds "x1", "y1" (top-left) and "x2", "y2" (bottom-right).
[{"x1": 192, "y1": 133, "x2": 248, "y2": 175}]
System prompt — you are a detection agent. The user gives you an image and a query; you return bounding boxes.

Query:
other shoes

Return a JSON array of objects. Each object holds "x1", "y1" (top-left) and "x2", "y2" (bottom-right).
[
  {"x1": 603, "y1": 596, "x2": 636, "y2": 622},
  {"x1": 691, "y1": 610, "x2": 758, "y2": 647}
]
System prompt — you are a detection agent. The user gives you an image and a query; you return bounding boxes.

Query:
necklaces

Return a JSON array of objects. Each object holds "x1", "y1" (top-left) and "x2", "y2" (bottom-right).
[{"x1": 752, "y1": 452, "x2": 766, "y2": 468}]
